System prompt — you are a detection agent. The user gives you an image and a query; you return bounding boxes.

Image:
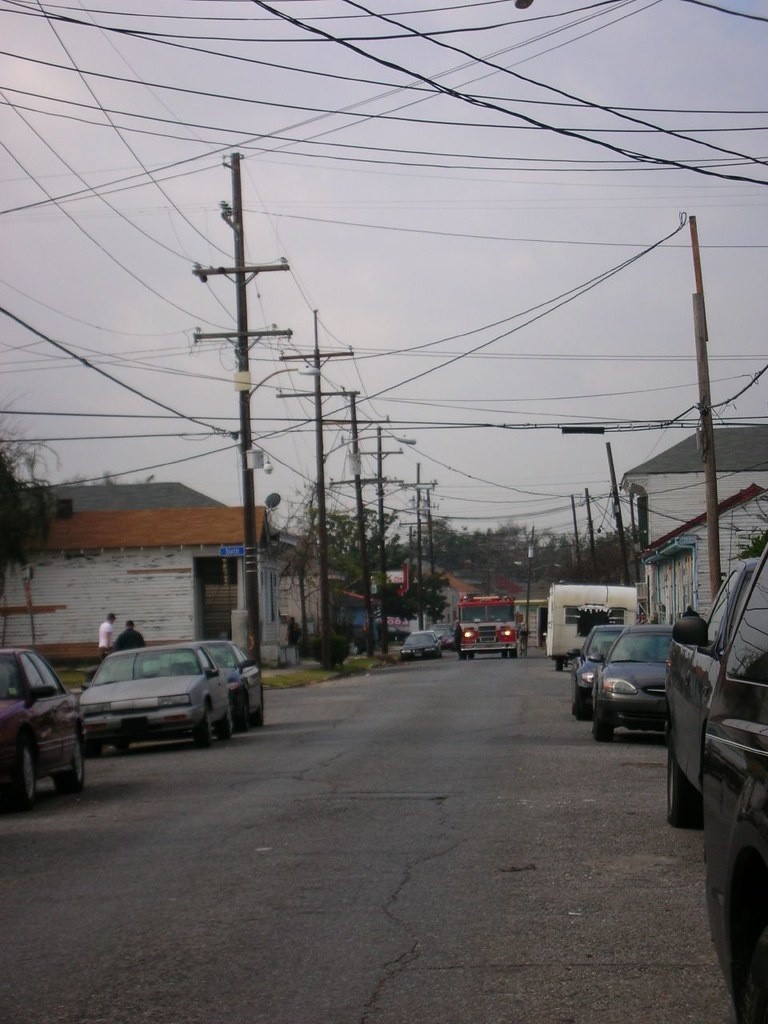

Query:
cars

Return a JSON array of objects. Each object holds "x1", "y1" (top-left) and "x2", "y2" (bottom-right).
[
  {"x1": 399, "y1": 630, "x2": 442, "y2": 661},
  {"x1": 587, "y1": 624, "x2": 674, "y2": 742},
  {"x1": 566, "y1": 625, "x2": 626, "y2": 722},
  {"x1": 176, "y1": 640, "x2": 264, "y2": 734},
  {"x1": 0, "y1": 648, "x2": 86, "y2": 813},
  {"x1": 79, "y1": 642, "x2": 233, "y2": 758},
  {"x1": 427, "y1": 626, "x2": 456, "y2": 651}
]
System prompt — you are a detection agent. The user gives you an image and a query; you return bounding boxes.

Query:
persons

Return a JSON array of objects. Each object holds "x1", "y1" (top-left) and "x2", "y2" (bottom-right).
[
  {"x1": 518, "y1": 623, "x2": 528, "y2": 657},
  {"x1": 116, "y1": 620, "x2": 146, "y2": 651},
  {"x1": 682, "y1": 606, "x2": 700, "y2": 617},
  {"x1": 97, "y1": 613, "x2": 117, "y2": 660},
  {"x1": 287, "y1": 616, "x2": 297, "y2": 646},
  {"x1": 452, "y1": 620, "x2": 462, "y2": 661}
]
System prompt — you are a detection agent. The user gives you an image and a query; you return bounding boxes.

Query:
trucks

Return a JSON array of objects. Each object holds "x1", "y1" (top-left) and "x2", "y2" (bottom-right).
[{"x1": 543, "y1": 583, "x2": 637, "y2": 672}]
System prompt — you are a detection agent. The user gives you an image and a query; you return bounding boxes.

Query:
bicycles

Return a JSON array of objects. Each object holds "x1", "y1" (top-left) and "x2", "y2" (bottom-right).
[{"x1": 518, "y1": 626, "x2": 529, "y2": 659}]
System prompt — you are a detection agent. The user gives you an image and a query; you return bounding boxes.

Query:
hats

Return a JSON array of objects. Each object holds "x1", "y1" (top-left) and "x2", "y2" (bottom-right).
[
  {"x1": 126, "y1": 621, "x2": 133, "y2": 626},
  {"x1": 107, "y1": 614, "x2": 116, "y2": 620}
]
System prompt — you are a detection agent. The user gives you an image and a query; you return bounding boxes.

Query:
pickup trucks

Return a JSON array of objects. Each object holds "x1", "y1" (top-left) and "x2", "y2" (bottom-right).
[{"x1": 664, "y1": 542, "x2": 767, "y2": 1024}]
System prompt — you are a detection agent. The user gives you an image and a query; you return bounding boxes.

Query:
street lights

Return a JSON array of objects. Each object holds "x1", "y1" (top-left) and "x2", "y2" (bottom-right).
[
  {"x1": 238, "y1": 364, "x2": 321, "y2": 667},
  {"x1": 378, "y1": 505, "x2": 431, "y2": 655},
  {"x1": 356, "y1": 483, "x2": 435, "y2": 657},
  {"x1": 317, "y1": 435, "x2": 417, "y2": 670}
]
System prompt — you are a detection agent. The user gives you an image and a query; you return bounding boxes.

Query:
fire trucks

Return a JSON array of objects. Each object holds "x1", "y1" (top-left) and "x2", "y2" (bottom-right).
[{"x1": 457, "y1": 592, "x2": 517, "y2": 660}]
